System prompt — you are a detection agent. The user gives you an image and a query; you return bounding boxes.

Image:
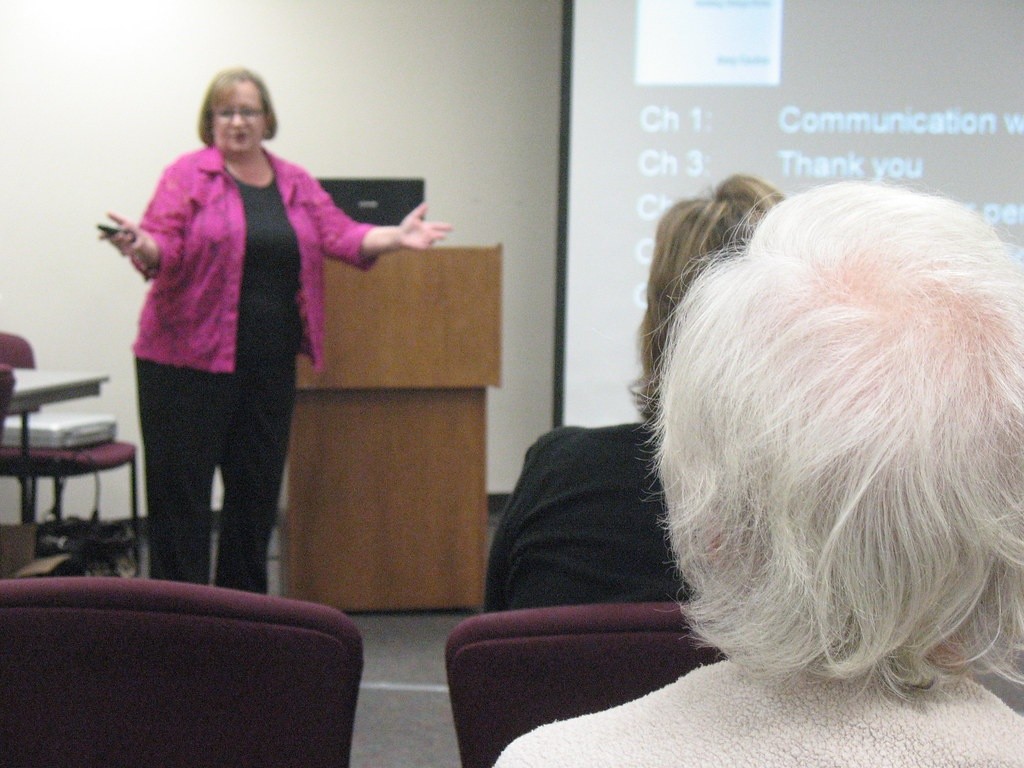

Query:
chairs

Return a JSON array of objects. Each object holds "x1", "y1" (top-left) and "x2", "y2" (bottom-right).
[
  {"x1": 0, "y1": 577, "x2": 363, "y2": 768},
  {"x1": 0, "y1": 332, "x2": 141, "y2": 577},
  {"x1": 442, "y1": 598, "x2": 722, "y2": 767}
]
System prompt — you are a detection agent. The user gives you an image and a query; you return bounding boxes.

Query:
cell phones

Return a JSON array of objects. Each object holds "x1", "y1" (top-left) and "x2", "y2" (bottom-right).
[{"x1": 98, "y1": 224, "x2": 135, "y2": 243}]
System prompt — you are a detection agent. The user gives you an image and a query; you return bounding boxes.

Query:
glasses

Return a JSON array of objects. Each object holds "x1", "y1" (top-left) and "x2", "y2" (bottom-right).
[{"x1": 214, "y1": 104, "x2": 266, "y2": 123}]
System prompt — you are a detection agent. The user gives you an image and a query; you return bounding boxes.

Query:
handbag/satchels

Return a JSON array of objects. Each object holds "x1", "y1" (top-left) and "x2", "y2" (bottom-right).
[{"x1": 36, "y1": 449, "x2": 141, "y2": 578}]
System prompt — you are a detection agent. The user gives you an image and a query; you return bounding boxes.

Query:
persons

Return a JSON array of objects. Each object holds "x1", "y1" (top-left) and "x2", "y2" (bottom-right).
[
  {"x1": 101, "y1": 69, "x2": 454, "y2": 595},
  {"x1": 490, "y1": 184, "x2": 1023, "y2": 767},
  {"x1": 484, "y1": 177, "x2": 783, "y2": 612}
]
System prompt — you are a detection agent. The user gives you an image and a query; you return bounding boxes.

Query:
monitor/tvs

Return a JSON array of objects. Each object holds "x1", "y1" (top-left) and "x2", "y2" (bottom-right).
[{"x1": 318, "y1": 178, "x2": 424, "y2": 228}]
